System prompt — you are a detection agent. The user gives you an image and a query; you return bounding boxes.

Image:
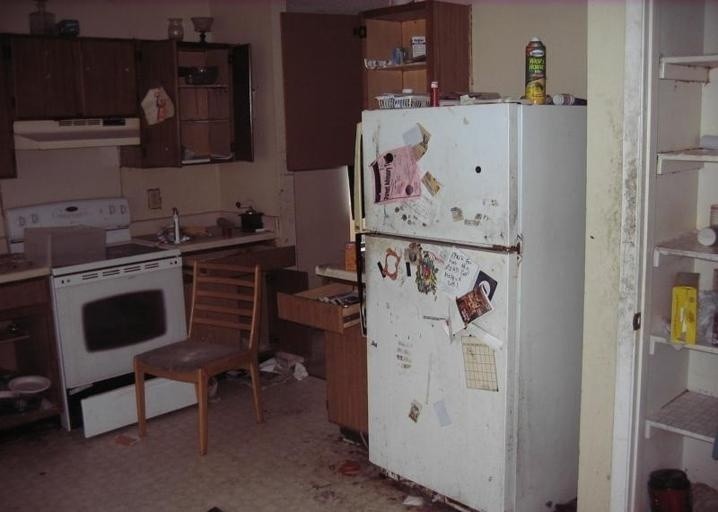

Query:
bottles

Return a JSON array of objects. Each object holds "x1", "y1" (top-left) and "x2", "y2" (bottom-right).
[
  {"x1": 695, "y1": 227, "x2": 717, "y2": 249},
  {"x1": 713, "y1": 267, "x2": 718, "y2": 293},
  {"x1": 429, "y1": 81, "x2": 440, "y2": 110},
  {"x1": 165, "y1": 16, "x2": 185, "y2": 41},
  {"x1": 711, "y1": 311, "x2": 717, "y2": 345},
  {"x1": 524, "y1": 35, "x2": 547, "y2": 105},
  {"x1": 551, "y1": 92, "x2": 577, "y2": 105},
  {"x1": 708, "y1": 203, "x2": 717, "y2": 227},
  {"x1": 172, "y1": 207, "x2": 183, "y2": 246},
  {"x1": 344, "y1": 241, "x2": 357, "y2": 272},
  {"x1": 29, "y1": 0, "x2": 56, "y2": 37}
]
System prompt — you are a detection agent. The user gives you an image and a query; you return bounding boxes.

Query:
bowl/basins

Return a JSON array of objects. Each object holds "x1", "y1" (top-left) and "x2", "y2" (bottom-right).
[
  {"x1": 2, "y1": 391, "x2": 42, "y2": 414},
  {"x1": 184, "y1": 64, "x2": 220, "y2": 85},
  {"x1": 238, "y1": 207, "x2": 265, "y2": 234}
]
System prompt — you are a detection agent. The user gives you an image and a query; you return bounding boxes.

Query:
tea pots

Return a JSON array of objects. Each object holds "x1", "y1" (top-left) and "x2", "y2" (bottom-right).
[{"x1": 391, "y1": 47, "x2": 406, "y2": 64}]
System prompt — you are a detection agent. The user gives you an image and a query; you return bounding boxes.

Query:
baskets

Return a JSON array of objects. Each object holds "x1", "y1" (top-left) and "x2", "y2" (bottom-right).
[{"x1": 377, "y1": 97, "x2": 430, "y2": 108}]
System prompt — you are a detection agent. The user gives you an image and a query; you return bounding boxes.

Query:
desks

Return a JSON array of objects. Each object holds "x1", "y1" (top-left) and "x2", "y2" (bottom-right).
[{"x1": 276, "y1": 265, "x2": 368, "y2": 441}]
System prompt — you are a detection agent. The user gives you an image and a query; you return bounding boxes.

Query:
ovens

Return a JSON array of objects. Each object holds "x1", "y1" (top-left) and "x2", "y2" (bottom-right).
[{"x1": 48, "y1": 257, "x2": 199, "y2": 440}]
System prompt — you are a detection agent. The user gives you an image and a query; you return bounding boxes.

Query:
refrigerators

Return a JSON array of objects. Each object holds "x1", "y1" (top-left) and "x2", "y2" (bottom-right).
[{"x1": 353, "y1": 105, "x2": 588, "y2": 511}]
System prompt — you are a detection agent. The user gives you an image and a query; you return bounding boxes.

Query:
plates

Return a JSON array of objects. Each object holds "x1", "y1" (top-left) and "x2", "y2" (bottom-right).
[{"x1": 6, "y1": 374, "x2": 53, "y2": 395}]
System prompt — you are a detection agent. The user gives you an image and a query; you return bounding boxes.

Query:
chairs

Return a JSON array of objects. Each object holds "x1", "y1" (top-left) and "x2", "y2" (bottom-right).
[{"x1": 134, "y1": 257, "x2": 264, "y2": 455}]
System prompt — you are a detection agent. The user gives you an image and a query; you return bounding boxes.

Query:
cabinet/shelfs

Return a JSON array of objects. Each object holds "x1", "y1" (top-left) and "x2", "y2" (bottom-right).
[
  {"x1": 0, "y1": 32, "x2": 254, "y2": 180},
  {"x1": 279, "y1": 0, "x2": 470, "y2": 173},
  {"x1": 610, "y1": 0, "x2": 718, "y2": 512},
  {"x1": 183, "y1": 241, "x2": 313, "y2": 356},
  {"x1": 0, "y1": 278, "x2": 63, "y2": 430}
]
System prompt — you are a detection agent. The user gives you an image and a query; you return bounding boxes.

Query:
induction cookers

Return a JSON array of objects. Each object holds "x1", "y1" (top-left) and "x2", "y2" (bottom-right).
[{"x1": 47, "y1": 238, "x2": 182, "y2": 277}]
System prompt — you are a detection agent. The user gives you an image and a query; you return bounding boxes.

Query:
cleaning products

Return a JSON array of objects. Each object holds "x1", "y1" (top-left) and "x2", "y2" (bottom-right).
[{"x1": 170, "y1": 207, "x2": 180, "y2": 243}]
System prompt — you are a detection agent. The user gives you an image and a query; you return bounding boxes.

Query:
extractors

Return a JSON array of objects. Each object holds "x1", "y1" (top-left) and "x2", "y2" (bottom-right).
[{"x1": 12, "y1": 116, "x2": 143, "y2": 152}]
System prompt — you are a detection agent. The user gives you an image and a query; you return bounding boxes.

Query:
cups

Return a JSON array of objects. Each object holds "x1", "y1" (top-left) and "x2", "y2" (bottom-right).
[
  {"x1": 363, "y1": 58, "x2": 377, "y2": 70},
  {"x1": 53, "y1": 19, "x2": 79, "y2": 37},
  {"x1": 646, "y1": 468, "x2": 689, "y2": 511},
  {"x1": 374, "y1": 58, "x2": 388, "y2": 69}
]
